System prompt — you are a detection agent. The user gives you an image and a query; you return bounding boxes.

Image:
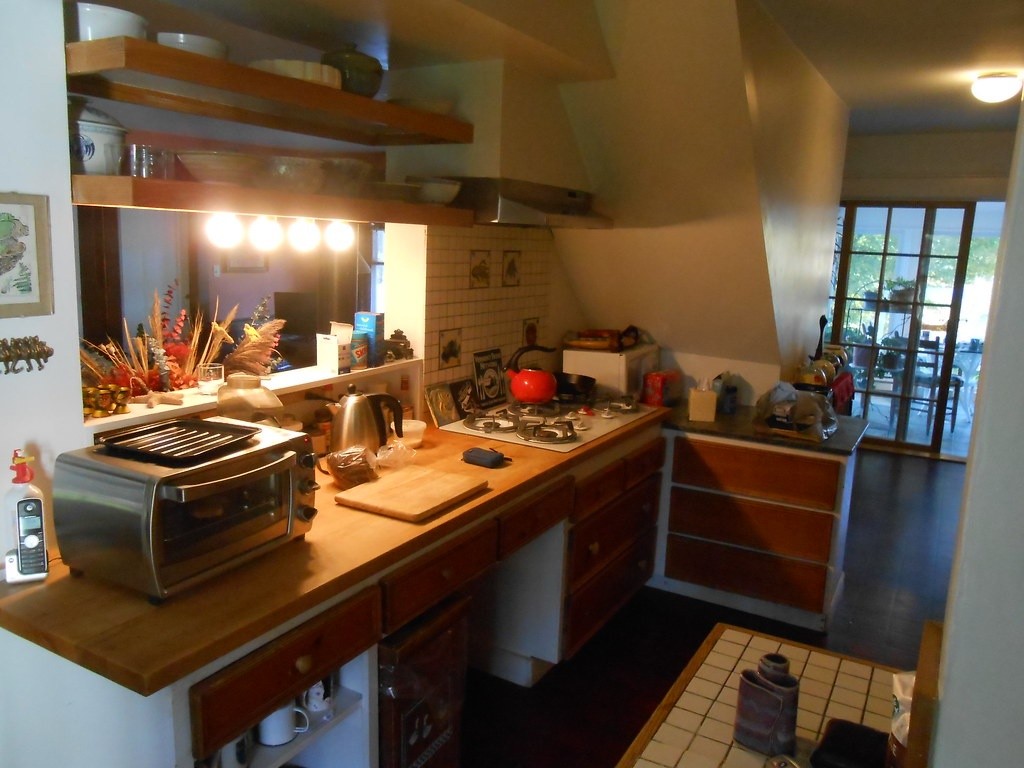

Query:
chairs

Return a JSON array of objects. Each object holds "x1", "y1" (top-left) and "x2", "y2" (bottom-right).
[{"x1": 892, "y1": 332, "x2": 964, "y2": 435}]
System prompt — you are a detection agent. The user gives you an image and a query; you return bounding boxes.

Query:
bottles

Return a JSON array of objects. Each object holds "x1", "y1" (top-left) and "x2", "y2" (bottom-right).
[
  {"x1": 380, "y1": 402, "x2": 389, "y2": 435},
  {"x1": 399, "y1": 378, "x2": 413, "y2": 420},
  {"x1": 282, "y1": 410, "x2": 333, "y2": 457},
  {"x1": 350, "y1": 331, "x2": 368, "y2": 368},
  {"x1": 6, "y1": 449, "x2": 45, "y2": 551}
]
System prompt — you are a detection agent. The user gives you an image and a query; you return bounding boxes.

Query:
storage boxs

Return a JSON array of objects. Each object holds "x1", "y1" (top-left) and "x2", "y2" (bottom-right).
[
  {"x1": 355, "y1": 311, "x2": 385, "y2": 368},
  {"x1": 317, "y1": 321, "x2": 354, "y2": 374}
]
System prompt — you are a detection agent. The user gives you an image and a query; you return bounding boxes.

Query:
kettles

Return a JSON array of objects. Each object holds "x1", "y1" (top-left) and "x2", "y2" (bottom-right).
[
  {"x1": 503, "y1": 346, "x2": 557, "y2": 405},
  {"x1": 326, "y1": 384, "x2": 403, "y2": 457}
]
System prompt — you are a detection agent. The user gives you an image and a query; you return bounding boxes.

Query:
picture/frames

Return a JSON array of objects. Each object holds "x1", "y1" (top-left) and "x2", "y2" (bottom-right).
[{"x1": 0, "y1": 193, "x2": 55, "y2": 319}]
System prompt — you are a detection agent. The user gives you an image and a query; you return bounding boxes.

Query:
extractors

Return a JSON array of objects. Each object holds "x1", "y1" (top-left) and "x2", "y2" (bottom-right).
[{"x1": 464, "y1": 180, "x2": 615, "y2": 229}]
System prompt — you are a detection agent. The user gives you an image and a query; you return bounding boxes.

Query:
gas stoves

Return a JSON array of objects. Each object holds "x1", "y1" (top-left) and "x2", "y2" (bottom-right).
[{"x1": 438, "y1": 392, "x2": 658, "y2": 453}]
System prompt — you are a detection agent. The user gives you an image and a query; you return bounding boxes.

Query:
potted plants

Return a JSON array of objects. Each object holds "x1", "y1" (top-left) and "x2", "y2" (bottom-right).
[{"x1": 846, "y1": 278, "x2": 914, "y2": 394}]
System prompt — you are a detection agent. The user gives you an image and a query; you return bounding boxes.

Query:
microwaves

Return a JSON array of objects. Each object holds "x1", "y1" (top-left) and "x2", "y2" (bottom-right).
[{"x1": 52, "y1": 412, "x2": 321, "y2": 608}]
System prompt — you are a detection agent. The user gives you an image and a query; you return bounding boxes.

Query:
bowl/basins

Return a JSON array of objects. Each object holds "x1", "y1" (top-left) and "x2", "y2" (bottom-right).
[
  {"x1": 177, "y1": 150, "x2": 461, "y2": 205},
  {"x1": 387, "y1": 98, "x2": 459, "y2": 121},
  {"x1": 66, "y1": 2, "x2": 224, "y2": 60},
  {"x1": 390, "y1": 419, "x2": 427, "y2": 449}
]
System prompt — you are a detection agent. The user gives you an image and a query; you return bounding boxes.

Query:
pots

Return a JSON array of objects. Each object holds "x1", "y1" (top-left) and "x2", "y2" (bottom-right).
[
  {"x1": 551, "y1": 373, "x2": 596, "y2": 396},
  {"x1": 67, "y1": 95, "x2": 129, "y2": 176},
  {"x1": 319, "y1": 43, "x2": 384, "y2": 98}
]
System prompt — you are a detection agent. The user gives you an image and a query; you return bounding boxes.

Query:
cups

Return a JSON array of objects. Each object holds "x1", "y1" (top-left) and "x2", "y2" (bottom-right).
[
  {"x1": 106, "y1": 143, "x2": 176, "y2": 182},
  {"x1": 198, "y1": 363, "x2": 224, "y2": 395},
  {"x1": 211, "y1": 673, "x2": 333, "y2": 768},
  {"x1": 368, "y1": 384, "x2": 386, "y2": 394}
]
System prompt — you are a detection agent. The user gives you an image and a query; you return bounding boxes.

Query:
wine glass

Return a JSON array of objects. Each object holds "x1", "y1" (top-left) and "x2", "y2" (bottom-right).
[{"x1": 82, "y1": 384, "x2": 131, "y2": 417}]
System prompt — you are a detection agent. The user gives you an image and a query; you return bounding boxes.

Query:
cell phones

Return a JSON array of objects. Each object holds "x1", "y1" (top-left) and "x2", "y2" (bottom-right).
[{"x1": 17, "y1": 497, "x2": 48, "y2": 575}]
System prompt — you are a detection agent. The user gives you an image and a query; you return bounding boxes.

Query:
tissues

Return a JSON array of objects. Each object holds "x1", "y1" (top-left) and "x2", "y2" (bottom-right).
[{"x1": 688, "y1": 376, "x2": 712, "y2": 421}]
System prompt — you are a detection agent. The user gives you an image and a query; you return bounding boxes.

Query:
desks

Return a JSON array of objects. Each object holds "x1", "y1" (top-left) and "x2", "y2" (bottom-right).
[{"x1": 614, "y1": 623, "x2": 942, "y2": 768}]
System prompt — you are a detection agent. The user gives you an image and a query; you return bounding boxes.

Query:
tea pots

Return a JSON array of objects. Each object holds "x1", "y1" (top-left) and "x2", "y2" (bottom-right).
[{"x1": 800, "y1": 345, "x2": 848, "y2": 387}]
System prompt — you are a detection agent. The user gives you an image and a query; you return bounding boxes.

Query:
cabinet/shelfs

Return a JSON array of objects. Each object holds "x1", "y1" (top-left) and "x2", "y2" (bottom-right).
[
  {"x1": 61, "y1": 0, "x2": 477, "y2": 226},
  {"x1": 0, "y1": 406, "x2": 669, "y2": 768},
  {"x1": 658, "y1": 407, "x2": 868, "y2": 633}
]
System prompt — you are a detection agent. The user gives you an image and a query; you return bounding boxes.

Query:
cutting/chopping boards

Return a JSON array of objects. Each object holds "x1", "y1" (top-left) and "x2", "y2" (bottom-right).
[{"x1": 335, "y1": 465, "x2": 488, "y2": 522}]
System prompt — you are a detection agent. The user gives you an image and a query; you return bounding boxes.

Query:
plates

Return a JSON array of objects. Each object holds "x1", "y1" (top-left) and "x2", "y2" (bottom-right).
[{"x1": 249, "y1": 60, "x2": 342, "y2": 91}]
